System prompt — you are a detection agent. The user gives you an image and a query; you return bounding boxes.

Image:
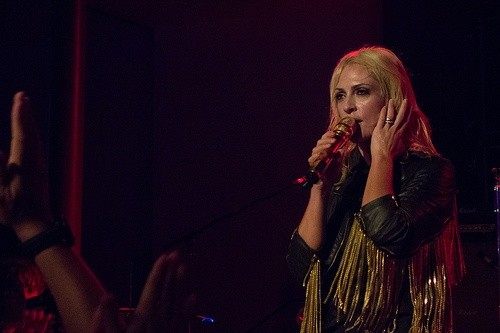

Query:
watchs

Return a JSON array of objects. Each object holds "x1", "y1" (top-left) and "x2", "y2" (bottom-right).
[{"x1": 10, "y1": 226, "x2": 74, "y2": 257}]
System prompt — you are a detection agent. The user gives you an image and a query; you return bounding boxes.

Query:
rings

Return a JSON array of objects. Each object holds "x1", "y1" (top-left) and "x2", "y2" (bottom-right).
[
  {"x1": 386, "y1": 117, "x2": 393, "y2": 120},
  {"x1": 386, "y1": 121, "x2": 393, "y2": 123}
]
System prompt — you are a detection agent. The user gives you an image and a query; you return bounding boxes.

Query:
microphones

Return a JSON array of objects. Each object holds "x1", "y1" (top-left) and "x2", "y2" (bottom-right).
[{"x1": 305, "y1": 117, "x2": 358, "y2": 183}]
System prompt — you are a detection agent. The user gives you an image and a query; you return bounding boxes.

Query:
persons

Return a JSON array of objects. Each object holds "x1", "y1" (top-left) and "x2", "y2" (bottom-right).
[
  {"x1": 296, "y1": 46, "x2": 459, "y2": 333},
  {"x1": 137, "y1": 248, "x2": 196, "y2": 333},
  {"x1": 0, "y1": 88, "x2": 117, "y2": 333}
]
